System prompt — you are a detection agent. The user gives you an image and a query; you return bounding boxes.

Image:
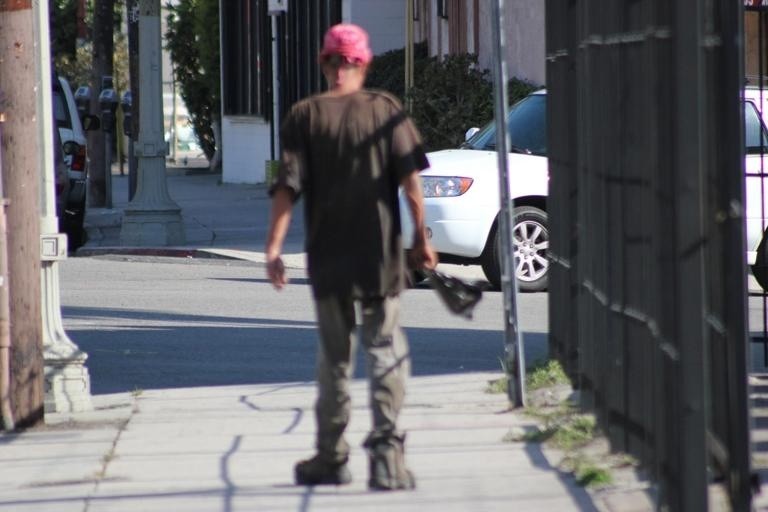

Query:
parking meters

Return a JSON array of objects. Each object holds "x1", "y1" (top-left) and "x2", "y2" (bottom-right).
[
  {"x1": 99, "y1": 89, "x2": 119, "y2": 210},
  {"x1": 74, "y1": 86, "x2": 89, "y2": 208},
  {"x1": 121, "y1": 90, "x2": 135, "y2": 202}
]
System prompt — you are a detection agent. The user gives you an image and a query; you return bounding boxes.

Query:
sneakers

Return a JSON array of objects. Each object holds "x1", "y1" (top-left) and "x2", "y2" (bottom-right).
[
  {"x1": 368, "y1": 444, "x2": 415, "y2": 489},
  {"x1": 295, "y1": 452, "x2": 351, "y2": 486}
]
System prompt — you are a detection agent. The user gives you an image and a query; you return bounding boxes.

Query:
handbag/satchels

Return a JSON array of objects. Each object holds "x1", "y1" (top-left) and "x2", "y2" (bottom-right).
[{"x1": 425, "y1": 268, "x2": 481, "y2": 315}]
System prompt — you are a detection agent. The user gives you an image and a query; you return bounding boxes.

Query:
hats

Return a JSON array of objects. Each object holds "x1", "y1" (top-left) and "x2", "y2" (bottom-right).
[{"x1": 319, "y1": 23, "x2": 374, "y2": 66}]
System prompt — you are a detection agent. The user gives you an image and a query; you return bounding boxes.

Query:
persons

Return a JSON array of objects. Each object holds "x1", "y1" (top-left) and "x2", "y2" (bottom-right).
[{"x1": 265, "y1": 24, "x2": 437, "y2": 492}]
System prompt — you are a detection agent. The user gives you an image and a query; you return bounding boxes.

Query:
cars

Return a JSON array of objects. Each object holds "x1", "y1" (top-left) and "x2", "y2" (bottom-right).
[
  {"x1": 398, "y1": 86, "x2": 767, "y2": 291},
  {"x1": 54, "y1": 76, "x2": 99, "y2": 211},
  {"x1": 54, "y1": 118, "x2": 71, "y2": 232}
]
